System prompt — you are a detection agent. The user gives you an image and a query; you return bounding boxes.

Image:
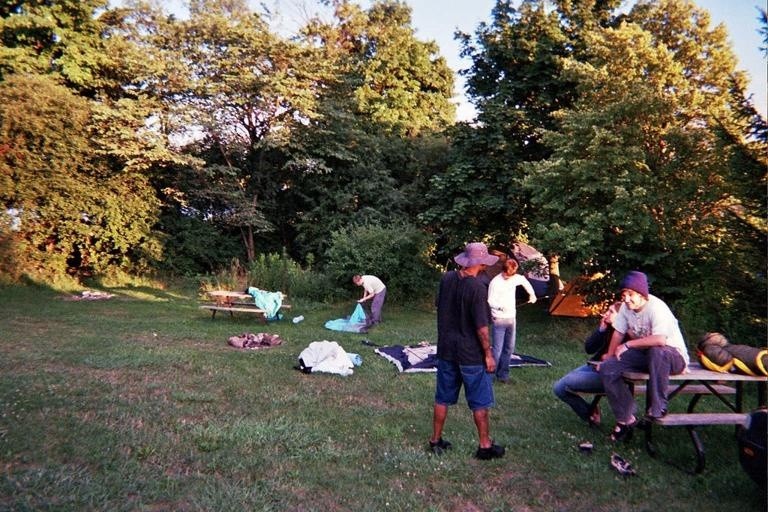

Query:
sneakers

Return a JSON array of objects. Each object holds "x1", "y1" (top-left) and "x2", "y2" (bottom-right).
[
  {"x1": 590, "y1": 403, "x2": 600, "y2": 428},
  {"x1": 579, "y1": 440, "x2": 636, "y2": 478},
  {"x1": 428, "y1": 437, "x2": 452, "y2": 451},
  {"x1": 476, "y1": 443, "x2": 505, "y2": 459}
]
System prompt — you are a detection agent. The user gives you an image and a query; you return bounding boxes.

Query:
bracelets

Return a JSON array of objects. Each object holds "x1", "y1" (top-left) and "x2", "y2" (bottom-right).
[{"x1": 624, "y1": 343, "x2": 631, "y2": 350}]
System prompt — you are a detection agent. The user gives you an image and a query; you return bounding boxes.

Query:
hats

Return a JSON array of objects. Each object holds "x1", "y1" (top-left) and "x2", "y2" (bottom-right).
[
  {"x1": 620, "y1": 270, "x2": 649, "y2": 299},
  {"x1": 453, "y1": 242, "x2": 500, "y2": 268}
]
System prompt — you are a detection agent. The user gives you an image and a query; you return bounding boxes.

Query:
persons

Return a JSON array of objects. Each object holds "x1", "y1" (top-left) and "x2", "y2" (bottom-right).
[
  {"x1": 486, "y1": 259, "x2": 538, "y2": 384},
  {"x1": 352, "y1": 274, "x2": 386, "y2": 325},
  {"x1": 598, "y1": 270, "x2": 691, "y2": 442},
  {"x1": 431, "y1": 242, "x2": 506, "y2": 461},
  {"x1": 553, "y1": 301, "x2": 634, "y2": 427}
]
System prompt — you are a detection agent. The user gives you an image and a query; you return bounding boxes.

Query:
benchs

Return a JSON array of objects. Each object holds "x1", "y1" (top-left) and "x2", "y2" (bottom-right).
[
  {"x1": 587, "y1": 385, "x2": 750, "y2": 472},
  {"x1": 198, "y1": 301, "x2": 292, "y2": 327}
]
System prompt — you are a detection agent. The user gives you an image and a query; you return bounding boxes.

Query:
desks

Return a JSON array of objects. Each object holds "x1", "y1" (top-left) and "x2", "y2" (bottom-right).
[
  {"x1": 622, "y1": 360, "x2": 768, "y2": 474},
  {"x1": 209, "y1": 291, "x2": 288, "y2": 326}
]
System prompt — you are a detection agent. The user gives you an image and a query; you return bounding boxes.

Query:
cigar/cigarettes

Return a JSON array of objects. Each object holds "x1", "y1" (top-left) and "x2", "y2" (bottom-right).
[{"x1": 600, "y1": 313, "x2": 608, "y2": 318}]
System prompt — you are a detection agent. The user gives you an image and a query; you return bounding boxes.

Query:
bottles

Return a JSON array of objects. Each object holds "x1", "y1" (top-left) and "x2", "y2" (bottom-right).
[{"x1": 292, "y1": 314, "x2": 304, "y2": 325}]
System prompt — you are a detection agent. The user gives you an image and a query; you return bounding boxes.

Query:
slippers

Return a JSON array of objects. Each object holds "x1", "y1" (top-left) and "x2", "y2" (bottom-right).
[
  {"x1": 609, "y1": 414, "x2": 640, "y2": 443},
  {"x1": 641, "y1": 407, "x2": 669, "y2": 423}
]
